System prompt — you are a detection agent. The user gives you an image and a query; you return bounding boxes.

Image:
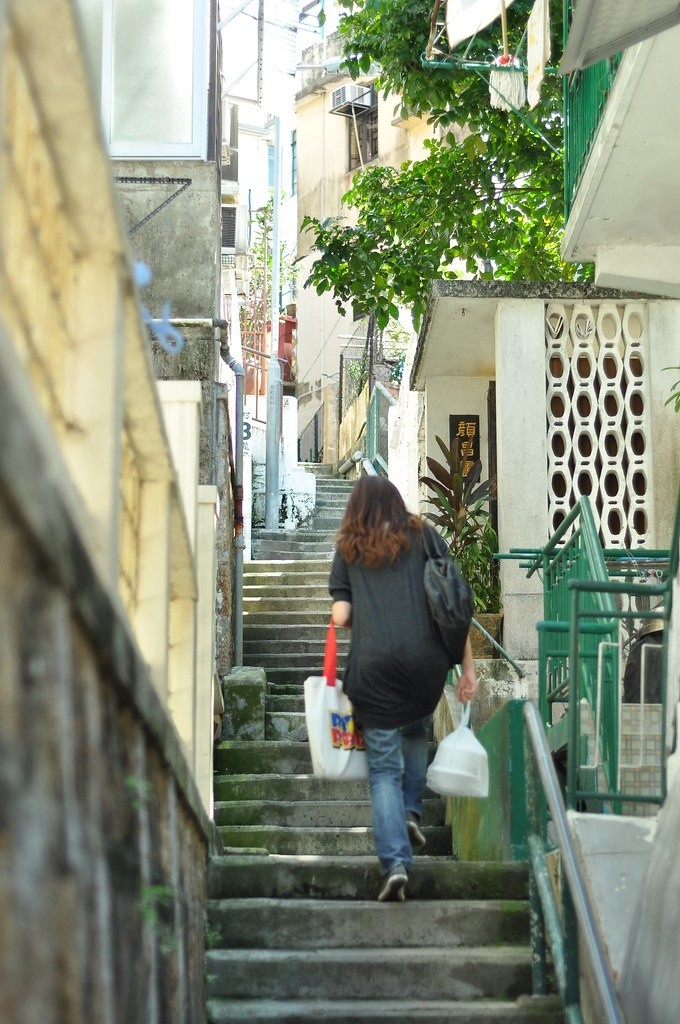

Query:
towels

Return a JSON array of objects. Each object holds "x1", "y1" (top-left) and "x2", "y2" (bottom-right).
[{"x1": 526, "y1": 0, "x2": 552, "y2": 107}]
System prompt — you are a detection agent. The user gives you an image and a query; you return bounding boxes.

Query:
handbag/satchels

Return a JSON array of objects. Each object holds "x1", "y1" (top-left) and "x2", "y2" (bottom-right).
[
  {"x1": 427, "y1": 699, "x2": 489, "y2": 797},
  {"x1": 304, "y1": 617, "x2": 369, "y2": 781},
  {"x1": 422, "y1": 521, "x2": 471, "y2": 664}
]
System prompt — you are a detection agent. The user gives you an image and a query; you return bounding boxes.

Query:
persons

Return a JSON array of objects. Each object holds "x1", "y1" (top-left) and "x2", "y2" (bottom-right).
[{"x1": 329, "y1": 476, "x2": 477, "y2": 903}]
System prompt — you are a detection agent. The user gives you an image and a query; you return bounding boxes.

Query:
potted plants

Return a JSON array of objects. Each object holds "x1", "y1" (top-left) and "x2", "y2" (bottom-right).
[{"x1": 420, "y1": 435, "x2": 502, "y2": 661}]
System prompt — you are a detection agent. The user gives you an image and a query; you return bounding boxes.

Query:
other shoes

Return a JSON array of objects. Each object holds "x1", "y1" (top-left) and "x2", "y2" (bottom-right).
[
  {"x1": 377, "y1": 865, "x2": 408, "y2": 901},
  {"x1": 405, "y1": 811, "x2": 425, "y2": 847}
]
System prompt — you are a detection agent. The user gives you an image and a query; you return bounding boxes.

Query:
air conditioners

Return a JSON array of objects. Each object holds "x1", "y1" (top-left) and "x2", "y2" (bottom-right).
[{"x1": 330, "y1": 84, "x2": 371, "y2": 110}]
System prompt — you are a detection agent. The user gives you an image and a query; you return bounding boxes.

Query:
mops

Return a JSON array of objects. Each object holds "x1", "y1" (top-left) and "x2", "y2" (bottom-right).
[{"x1": 488, "y1": 0, "x2": 526, "y2": 113}]
